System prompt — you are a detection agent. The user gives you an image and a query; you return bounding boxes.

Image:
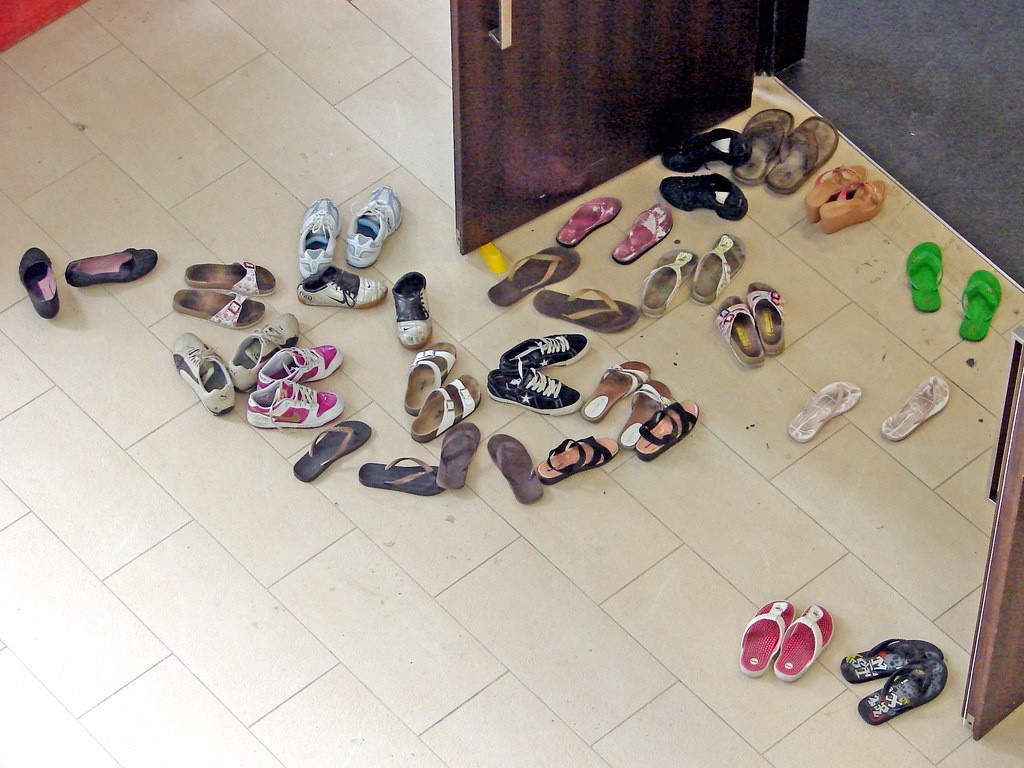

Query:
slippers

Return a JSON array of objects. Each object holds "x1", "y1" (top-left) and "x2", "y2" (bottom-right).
[
  {"x1": 789, "y1": 382, "x2": 860, "y2": 444},
  {"x1": 880, "y1": 377, "x2": 950, "y2": 441},
  {"x1": 487, "y1": 197, "x2": 1001, "y2": 368},
  {"x1": 291, "y1": 345, "x2": 700, "y2": 504},
  {"x1": 731, "y1": 109, "x2": 839, "y2": 194},
  {"x1": 172, "y1": 289, "x2": 266, "y2": 330},
  {"x1": 184, "y1": 261, "x2": 277, "y2": 297},
  {"x1": 739, "y1": 600, "x2": 948, "y2": 726}
]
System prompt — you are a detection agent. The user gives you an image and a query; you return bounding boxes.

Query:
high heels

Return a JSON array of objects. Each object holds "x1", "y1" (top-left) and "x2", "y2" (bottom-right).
[{"x1": 804, "y1": 165, "x2": 889, "y2": 234}]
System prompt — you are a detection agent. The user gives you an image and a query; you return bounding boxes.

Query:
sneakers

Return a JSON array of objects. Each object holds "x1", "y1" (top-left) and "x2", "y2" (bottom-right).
[
  {"x1": 298, "y1": 197, "x2": 341, "y2": 279},
  {"x1": 486, "y1": 332, "x2": 590, "y2": 416},
  {"x1": 256, "y1": 344, "x2": 343, "y2": 390},
  {"x1": 392, "y1": 271, "x2": 433, "y2": 351},
  {"x1": 344, "y1": 186, "x2": 402, "y2": 269},
  {"x1": 296, "y1": 264, "x2": 388, "y2": 310},
  {"x1": 246, "y1": 379, "x2": 345, "y2": 432},
  {"x1": 227, "y1": 313, "x2": 299, "y2": 393},
  {"x1": 173, "y1": 332, "x2": 236, "y2": 416},
  {"x1": 659, "y1": 128, "x2": 753, "y2": 222}
]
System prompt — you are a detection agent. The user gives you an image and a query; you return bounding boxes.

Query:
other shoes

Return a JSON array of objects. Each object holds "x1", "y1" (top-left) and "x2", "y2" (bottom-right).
[
  {"x1": 19, "y1": 247, "x2": 60, "y2": 320},
  {"x1": 65, "y1": 248, "x2": 158, "y2": 287}
]
